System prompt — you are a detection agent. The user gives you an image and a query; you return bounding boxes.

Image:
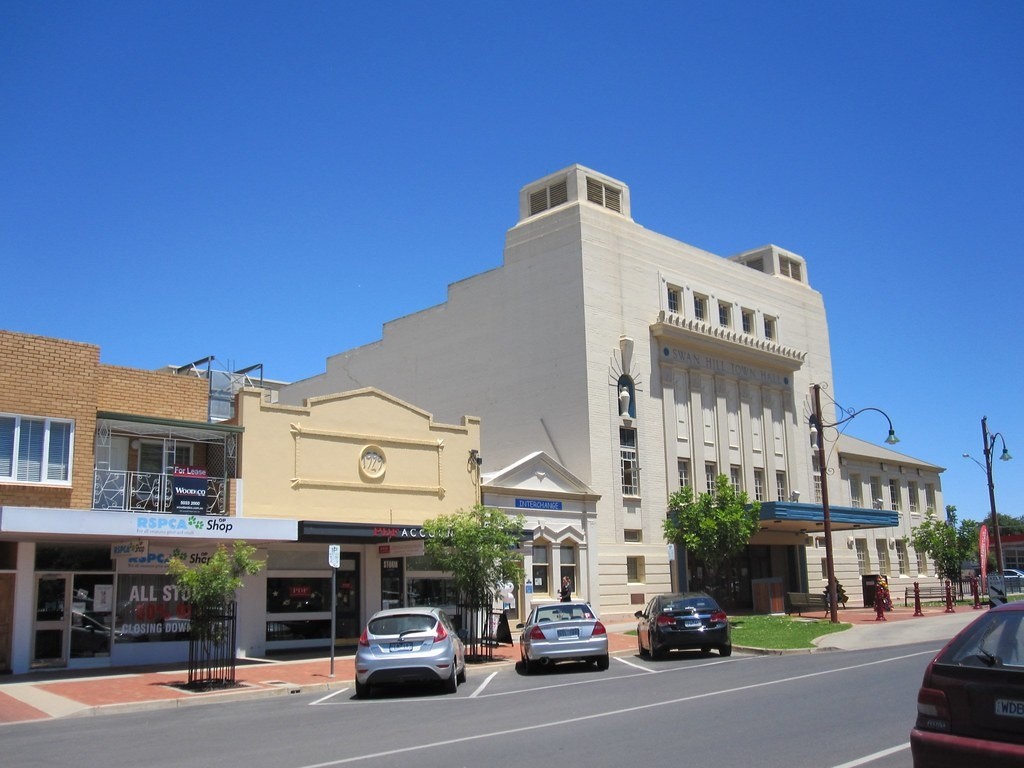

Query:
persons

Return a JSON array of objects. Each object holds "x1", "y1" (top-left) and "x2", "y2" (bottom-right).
[{"x1": 557, "y1": 576, "x2": 573, "y2": 617}]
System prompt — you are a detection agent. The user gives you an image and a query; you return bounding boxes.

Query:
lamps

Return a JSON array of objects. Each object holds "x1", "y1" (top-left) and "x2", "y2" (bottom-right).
[{"x1": 789, "y1": 490, "x2": 801, "y2": 503}]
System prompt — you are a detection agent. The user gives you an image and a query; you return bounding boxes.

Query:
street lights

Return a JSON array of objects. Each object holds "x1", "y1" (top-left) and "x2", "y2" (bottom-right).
[
  {"x1": 963, "y1": 415, "x2": 1014, "y2": 602},
  {"x1": 808, "y1": 382, "x2": 901, "y2": 626}
]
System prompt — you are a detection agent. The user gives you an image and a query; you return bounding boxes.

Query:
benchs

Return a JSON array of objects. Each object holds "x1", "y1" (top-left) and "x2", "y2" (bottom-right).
[{"x1": 783, "y1": 592, "x2": 829, "y2": 618}]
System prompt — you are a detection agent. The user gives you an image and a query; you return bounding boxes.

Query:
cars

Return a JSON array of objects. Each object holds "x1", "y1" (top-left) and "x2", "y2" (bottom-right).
[
  {"x1": 635, "y1": 592, "x2": 733, "y2": 660},
  {"x1": 352, "y1": 606, "x2": 470, "y2": 693},
  {"x1": 909, "y1": 599, "x2": 1024, "y2": 768},
  {"x1": 979, "y1": 568, "x2": 1024, "y2": 588},
  {"x1": 37, "y1": 609, "x2": 130, "y2": 655},
  {"x1": 515, "y1": 601, "x2": 610, "y2": 670}
]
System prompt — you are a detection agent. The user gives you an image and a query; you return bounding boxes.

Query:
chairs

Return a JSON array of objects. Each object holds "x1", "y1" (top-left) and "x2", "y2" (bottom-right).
[
  {"x1": 571, "y1": 616, "x2": 582, "y2": 619},
  {"x1": 539, "y1": 618, "x2": 551, "y2": 622}
]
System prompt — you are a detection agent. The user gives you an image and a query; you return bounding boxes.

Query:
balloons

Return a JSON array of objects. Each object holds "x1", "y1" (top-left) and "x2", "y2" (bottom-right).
[{"x1": 501, "y1": 582, "x2": 514, "y2": 603}]
